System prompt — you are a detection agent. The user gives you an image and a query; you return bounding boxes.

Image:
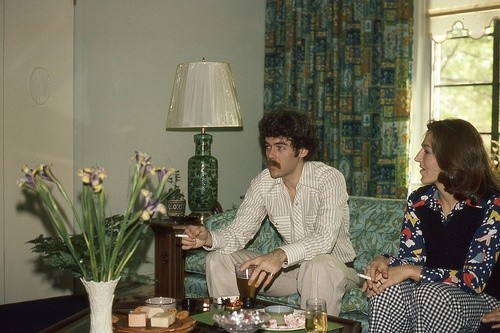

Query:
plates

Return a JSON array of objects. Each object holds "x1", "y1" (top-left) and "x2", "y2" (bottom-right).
[{"x1": 254, "y1": 308, "x2": 306, "y2": 332}]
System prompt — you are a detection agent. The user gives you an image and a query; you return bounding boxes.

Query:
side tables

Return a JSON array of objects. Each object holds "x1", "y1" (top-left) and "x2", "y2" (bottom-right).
[{"x1": 144, "y1": 217, "x2": 203, "y2": 303}]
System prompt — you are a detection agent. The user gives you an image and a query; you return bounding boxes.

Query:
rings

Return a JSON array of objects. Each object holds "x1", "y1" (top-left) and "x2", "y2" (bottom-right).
[
  {"x1": 269, "y1": 273, "x2": 274, "y2": 277},
  {"x1": 378, "y1": 280, "x2": 383, "y2": 285},
  {"x1": 261, "y1": 270, "x2": 268, "y2": 274}
]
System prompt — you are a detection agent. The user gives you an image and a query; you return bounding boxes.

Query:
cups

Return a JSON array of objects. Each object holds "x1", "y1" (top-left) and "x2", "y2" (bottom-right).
[
  {"x1": 304, "y1": 298, "x2": 328, "y2": 333},
  {"x1": 234, "y1": 262, "x2": 262, "y2": 309}
]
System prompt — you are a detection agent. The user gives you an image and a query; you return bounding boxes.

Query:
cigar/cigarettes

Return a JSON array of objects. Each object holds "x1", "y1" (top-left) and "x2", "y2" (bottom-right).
[
  {"x1": 175, "y1": 234, "x2": 188, "y2": 237},
  {"x1": 356, "y1": 273, "x2": 371, "y2": 281}
]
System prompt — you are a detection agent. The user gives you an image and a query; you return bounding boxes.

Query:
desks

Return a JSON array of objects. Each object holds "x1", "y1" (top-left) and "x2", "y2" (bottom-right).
[{"x1": 39, "y1": 296, "x2": 361, "y2": 333}]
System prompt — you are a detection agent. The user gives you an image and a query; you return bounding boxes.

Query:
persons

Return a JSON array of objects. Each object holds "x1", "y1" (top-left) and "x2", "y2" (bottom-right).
[
  {"x1": 181, "y1": 109, "x2": 360, "y2": 317},
  {"x1": 361, "y1": 118, "x2": 500, "y2": 333}
]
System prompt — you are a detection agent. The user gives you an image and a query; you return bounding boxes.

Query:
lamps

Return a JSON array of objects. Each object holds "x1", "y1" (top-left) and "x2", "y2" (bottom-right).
[{"x1": 166, "y1": 57, "x2": 243, "y2": 219}]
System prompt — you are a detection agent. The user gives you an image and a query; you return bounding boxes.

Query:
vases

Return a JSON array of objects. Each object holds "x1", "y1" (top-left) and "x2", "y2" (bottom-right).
[{"x1": 80, "y1": 275, "x2": 122, "y2": 333}]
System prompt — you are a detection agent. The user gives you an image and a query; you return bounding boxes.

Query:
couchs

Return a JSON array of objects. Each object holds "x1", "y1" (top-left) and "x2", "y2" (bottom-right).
[{"x1": 183, "y1": 195, "x2": 409, "y2": 333}]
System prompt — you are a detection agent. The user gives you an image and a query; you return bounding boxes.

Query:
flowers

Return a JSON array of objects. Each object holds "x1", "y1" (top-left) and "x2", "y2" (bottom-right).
[{"x1": 15, "y1": 150, "x2": 180, "y2": 283}]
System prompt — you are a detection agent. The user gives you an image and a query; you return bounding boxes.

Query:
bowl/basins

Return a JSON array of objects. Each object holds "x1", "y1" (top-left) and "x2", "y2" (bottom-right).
[
  {"x1": 211, "y1": 310, "x2": 271, "y2": 333},
  {"x1": 145, "y1": 297, "x2": 176, "y2": 309}
]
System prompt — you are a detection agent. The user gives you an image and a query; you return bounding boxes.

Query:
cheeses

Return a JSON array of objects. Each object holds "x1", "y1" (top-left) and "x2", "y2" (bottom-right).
[{"x1": 128, "y1": 306, "x2": 177, "y2": 328}]
